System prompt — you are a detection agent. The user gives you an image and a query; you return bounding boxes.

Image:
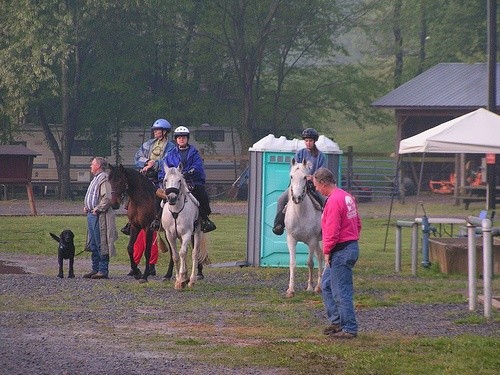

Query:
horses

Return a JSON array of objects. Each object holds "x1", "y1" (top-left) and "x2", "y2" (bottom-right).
[
  {"x1": 284, "y1": 156, "x2": 328, "y2": 300},
  {"x1": 161, "y1": 162, "x2": 212, "y2": 290},
  {"x1": 108, "y1": 163, "x2": 174, "y2": 285}
]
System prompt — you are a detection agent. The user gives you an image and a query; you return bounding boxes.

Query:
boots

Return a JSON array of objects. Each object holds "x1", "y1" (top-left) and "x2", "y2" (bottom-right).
[{"x1": 143, "y1": 264, "x2": 155, "y2": 276}]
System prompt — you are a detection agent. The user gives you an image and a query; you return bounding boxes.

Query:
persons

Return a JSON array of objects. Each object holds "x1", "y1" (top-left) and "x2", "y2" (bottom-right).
[
  {"x1": 122, "y1": 118, "x2": 176, "y2": 235},
  {"x1": 127, "y1": 226, "x2": 158, "y2": 278},
  {"x1": 312, "y1": 167, "x2": 362, "y2": 338},
  {"x1": 272, "y1": 128, "x2": 328, "y2": 235},
  {"x1": 82, "y1": 156, "x2": 118, "y2": 279},
  {"x1": 158, "y1": 126, "x2": 214, "y2": 232}
]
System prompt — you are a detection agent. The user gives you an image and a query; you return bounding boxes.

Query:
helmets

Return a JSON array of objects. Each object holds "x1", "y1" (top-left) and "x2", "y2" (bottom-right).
[
  {"x1": 302, "y1": 128, "x2": 318, "y2": 142},
  {"x1": 151, "y1": 118, "x2": 172, "y2": 133},
  {"x1": 174, "y1": 126, "x2": 190, "y2": 139}
]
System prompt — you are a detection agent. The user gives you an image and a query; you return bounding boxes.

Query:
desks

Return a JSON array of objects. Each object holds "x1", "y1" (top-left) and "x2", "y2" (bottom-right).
[{"x1": 414, "y1": 216, "x2": 466, "y2": 254}]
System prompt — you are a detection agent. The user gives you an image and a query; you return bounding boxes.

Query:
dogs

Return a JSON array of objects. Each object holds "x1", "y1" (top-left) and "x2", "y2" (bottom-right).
[{"x1": 49, "y1": 230, "x2": 76, "y2": 279}]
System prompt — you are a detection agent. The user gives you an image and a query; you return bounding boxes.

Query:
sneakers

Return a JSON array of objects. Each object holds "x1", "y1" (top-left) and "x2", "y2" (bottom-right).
[
  {"x1": 273, "y1": 212, "x2": 285, "y2": 235},
  {"x1": 91, "y1": 272, "x2": 108, "y2": 278},
  {"x1": 322, "y1": 324, "x2": 341, "y2": 334},
  {"x1": 330, "y1": 330, "x2": 358, "y2": 339},
  {"x1": 121, "y1": 222, "x2": 131, "y2": 234},
  {"x1": 84, "y1": 271, "x2": 97, "y2": 278}
]
